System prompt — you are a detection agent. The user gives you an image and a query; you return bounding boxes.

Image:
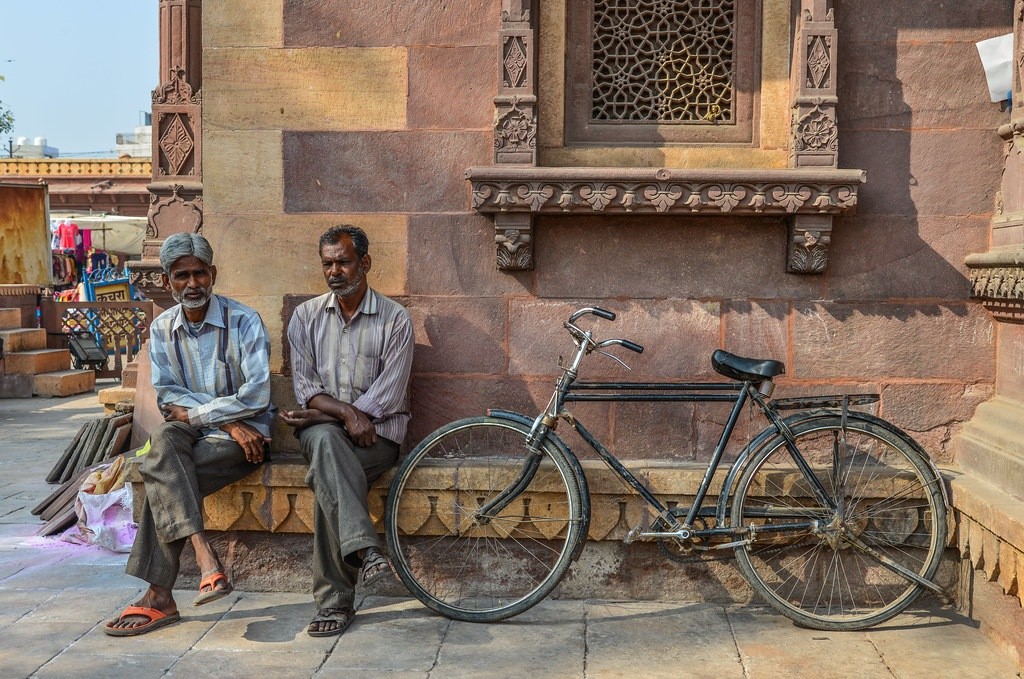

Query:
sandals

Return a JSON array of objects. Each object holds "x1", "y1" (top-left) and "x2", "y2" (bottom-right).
[
  {"x1": 308, "y1": 606, "x2": 356, "y2": 637},
  {"x1": 361, "y1": 546, "x2": 392, "y2": 588}
]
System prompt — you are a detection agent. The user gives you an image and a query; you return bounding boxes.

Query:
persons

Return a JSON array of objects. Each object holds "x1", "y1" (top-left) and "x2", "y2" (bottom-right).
[
  {"x1": 103, "y1": 231, "x2": 278, "y2": 635},
  {"x1": 279, "y1": 225, "x2": 415, "y2": 637}
]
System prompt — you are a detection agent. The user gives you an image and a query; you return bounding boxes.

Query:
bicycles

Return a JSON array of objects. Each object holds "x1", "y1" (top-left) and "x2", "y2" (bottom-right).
[{"x1": 385, "y1": 308, "x2": 954, "y2": 632}]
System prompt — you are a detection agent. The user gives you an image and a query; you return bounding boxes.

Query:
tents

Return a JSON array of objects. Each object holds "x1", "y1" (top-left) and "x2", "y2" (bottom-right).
[{"x1": 50, "y1": 218, "x2": 148, "y2": 254}]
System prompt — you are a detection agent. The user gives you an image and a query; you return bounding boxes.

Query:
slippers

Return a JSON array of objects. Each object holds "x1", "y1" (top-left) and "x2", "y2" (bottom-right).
[
  {"x1": 104, "y1": 605, "x2": 180, "y2": 636},
  {"x1": 193, "y1": 573, "x2": 233, "y2": 606}
]
